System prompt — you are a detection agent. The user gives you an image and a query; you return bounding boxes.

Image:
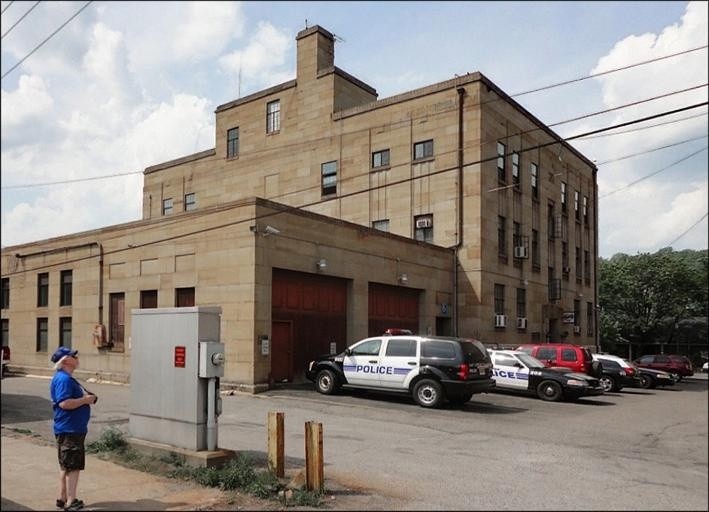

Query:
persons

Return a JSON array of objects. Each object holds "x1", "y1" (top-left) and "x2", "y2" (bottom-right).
[{"x1": 49, "y1": 346, "x2": 96, "y2": 511}]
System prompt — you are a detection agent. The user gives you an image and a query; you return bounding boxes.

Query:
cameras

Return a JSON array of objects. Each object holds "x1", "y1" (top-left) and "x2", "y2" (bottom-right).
[{"x1": 88, "y1": 392, "x2": 98, "y2": 404}]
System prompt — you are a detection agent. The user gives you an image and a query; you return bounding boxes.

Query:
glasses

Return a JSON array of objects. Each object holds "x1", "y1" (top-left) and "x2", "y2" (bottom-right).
[{"x1": 71, "y1": 355, "x2": 75, "y2": 358}]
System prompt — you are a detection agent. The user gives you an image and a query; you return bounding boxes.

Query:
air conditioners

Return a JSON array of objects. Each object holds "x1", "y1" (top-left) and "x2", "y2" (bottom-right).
[
  {"x1": 517, "y1": 318, "x2": 528, "y2": 329},
  {"x1": 515, "y1": 246, "x2": 529, "y2": 258},
  {"x1": 566, "y1": 267, "x2": 570, "y2": 272},
  {"x1": 494, "y1": 314, "x2": 508, "y2": 327},
  {"x1": 416, "y1": 220, "x2": 430, "y2": 228},
  {"x1": 574, "y1": 326, "x2": 579, "y2": 332}
]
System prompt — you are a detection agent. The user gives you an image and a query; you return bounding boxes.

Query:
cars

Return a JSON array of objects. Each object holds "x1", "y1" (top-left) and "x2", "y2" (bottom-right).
[
  {"x1": 592, "y1": 353, "x2": 680, "y2": 393},
  {"x1": 485, "y1": 346, "x2": 603, "y2": 400}
]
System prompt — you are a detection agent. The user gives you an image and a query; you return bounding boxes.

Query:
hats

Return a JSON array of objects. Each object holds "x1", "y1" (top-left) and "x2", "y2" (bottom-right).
[{"x1": 50, "y1": 347, "x2": 78, "y2": 363}]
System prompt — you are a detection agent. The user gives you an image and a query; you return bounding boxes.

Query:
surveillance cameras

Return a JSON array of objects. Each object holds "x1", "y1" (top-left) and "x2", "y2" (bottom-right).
[{"x1": 265, "y1": 226, "x2": 280, "y2": 235}]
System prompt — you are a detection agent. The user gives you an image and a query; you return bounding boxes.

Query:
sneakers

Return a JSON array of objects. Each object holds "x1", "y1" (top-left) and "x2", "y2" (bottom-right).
[{"x1": 55, "y1": 498, "x2": 84, "y2": 511}]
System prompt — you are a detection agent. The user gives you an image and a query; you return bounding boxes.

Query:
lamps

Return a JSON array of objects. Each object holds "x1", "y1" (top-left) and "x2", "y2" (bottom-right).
[
  {"x1": 316, "y1": 259, "x2": 327, "y2": 271},
  {"x1": 396, "y1": 272, "x2": 409, "y2": 284}
]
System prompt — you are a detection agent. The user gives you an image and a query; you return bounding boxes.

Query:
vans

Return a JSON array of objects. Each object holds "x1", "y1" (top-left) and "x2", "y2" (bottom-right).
[
  {"x1": 514, "y1": 344, "x2": 594, "y2": 374},
  {"x1": 305, "y1": 328, "x2": 496, "y2": 407},
  {"x1": 635, "y1": 354, "x2": 694, "y2": 382}
]
